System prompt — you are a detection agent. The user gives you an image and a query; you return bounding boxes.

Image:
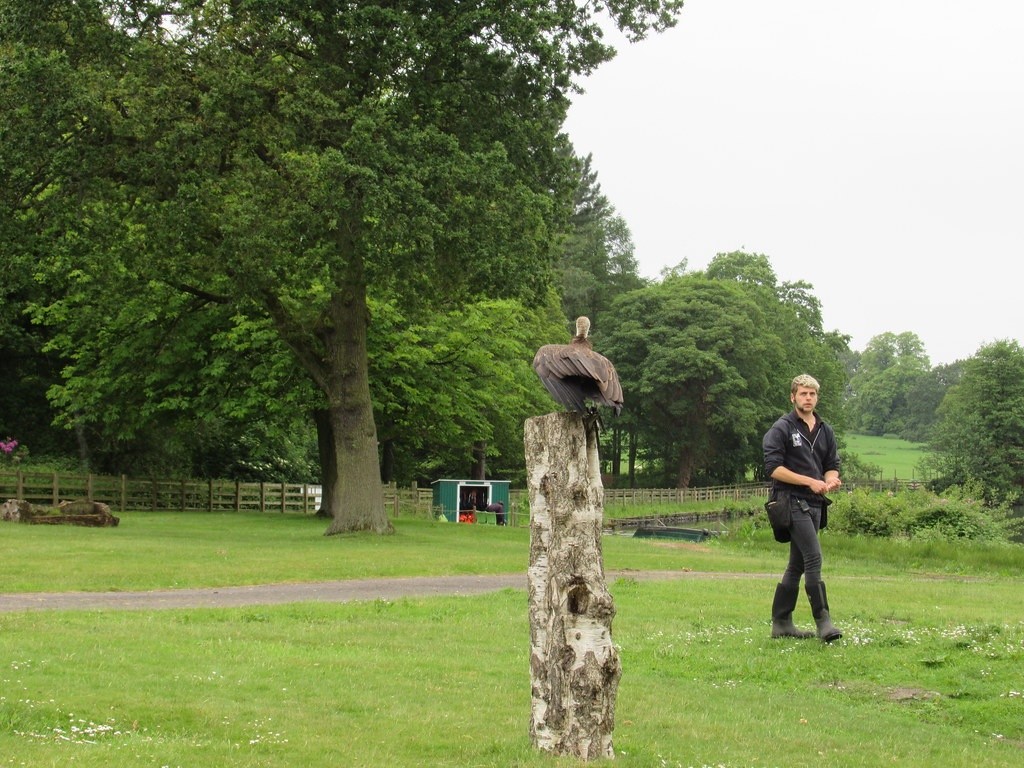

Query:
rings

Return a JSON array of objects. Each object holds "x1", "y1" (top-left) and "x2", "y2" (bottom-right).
[{"x1": 832, "y1": 489, "x2": 833, "y2": 490}]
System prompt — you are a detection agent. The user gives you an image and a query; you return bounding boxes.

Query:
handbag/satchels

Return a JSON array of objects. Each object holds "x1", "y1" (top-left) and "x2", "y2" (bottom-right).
[{"x1": 764, "y1": 491, "x2": 793, "y2": 544}]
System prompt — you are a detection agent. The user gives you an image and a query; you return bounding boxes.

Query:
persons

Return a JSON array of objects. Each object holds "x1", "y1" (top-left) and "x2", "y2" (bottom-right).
[
  {"x1": 763, "y1": 372, "x2": 843, "y2": 641},
  {"x1": 487, "y1": 501, "x2": 504, "y2": 526}
]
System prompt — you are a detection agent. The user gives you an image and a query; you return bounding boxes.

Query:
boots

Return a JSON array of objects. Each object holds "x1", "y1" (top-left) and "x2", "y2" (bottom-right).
[
  {"x1": 771, "y1": 582, "x2": 815, "y2": 638},
  {"x1": 805, "y1": 581, "x2": 843, "y2": 642}
]
